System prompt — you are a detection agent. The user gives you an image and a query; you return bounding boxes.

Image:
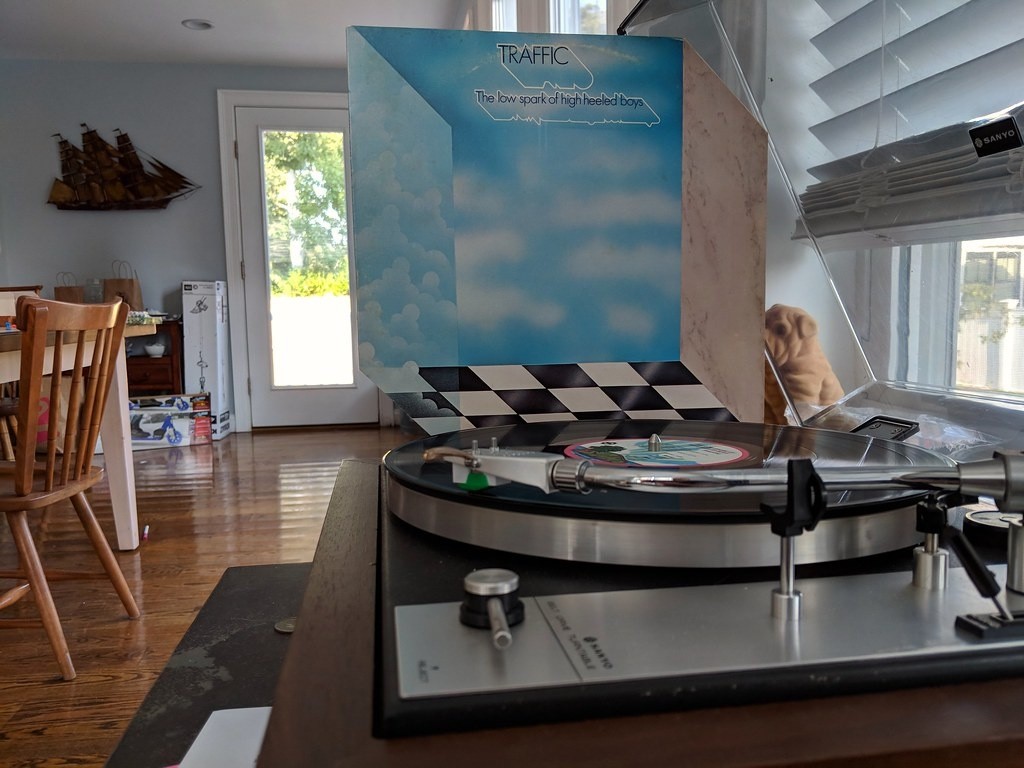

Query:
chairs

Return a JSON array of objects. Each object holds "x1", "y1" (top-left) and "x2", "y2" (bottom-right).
[{"x1": 0, "y1": 291, "x2": 140, "y2": 679}]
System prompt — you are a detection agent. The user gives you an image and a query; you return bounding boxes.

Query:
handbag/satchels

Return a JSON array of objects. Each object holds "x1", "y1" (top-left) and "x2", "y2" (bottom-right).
[
  {"x1": 54, "y1": 271, "x2": 84, "y2": 304},
  {"x1": 36, "y1": 376, "x2": 86, "y2": 453},
  {"x1": 103, "y1": 260, "x2": 143, "y2": 311}
]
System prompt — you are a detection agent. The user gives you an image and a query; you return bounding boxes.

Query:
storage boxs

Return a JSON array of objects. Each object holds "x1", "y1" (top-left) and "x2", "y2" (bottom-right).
[
  {"x1": 182, "y1": 279, "x2": 233, "y2": 441},
  {"x1": 95, "y1": 395, "x2": 213, "y2": 455}
]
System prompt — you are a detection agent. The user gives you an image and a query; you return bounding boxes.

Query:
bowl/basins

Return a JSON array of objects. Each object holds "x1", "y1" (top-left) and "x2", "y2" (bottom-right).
[{"x1": 145, "y1": 345, "x2": 166, "y2": 358}]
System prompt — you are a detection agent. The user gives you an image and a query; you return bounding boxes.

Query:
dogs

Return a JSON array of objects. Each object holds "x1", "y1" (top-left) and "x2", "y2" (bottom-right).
[{"x1": 763, "y1": 304, "x2": 846, "y2": 424}]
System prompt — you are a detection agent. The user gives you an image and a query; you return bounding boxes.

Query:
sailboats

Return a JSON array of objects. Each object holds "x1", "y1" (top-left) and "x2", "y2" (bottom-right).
[{"x1": 45, "y1": 123, "x2": 203, "y2": 212}]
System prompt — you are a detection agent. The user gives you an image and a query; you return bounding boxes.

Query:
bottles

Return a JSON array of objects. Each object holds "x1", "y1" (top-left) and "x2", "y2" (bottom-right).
[{"x1": 86, "y1": 277, "x2": 103, "y2": 304}]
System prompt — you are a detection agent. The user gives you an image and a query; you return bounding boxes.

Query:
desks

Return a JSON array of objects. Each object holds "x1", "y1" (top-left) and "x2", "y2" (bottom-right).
[{"x1": 0, "y1": 323, "x2": 156, "y2": 552}]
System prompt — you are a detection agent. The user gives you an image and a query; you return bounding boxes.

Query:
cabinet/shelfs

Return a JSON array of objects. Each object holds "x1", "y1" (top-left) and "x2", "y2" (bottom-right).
[{"x1": 125, "y1": 320, "x2": 181, "y2": 398}]
